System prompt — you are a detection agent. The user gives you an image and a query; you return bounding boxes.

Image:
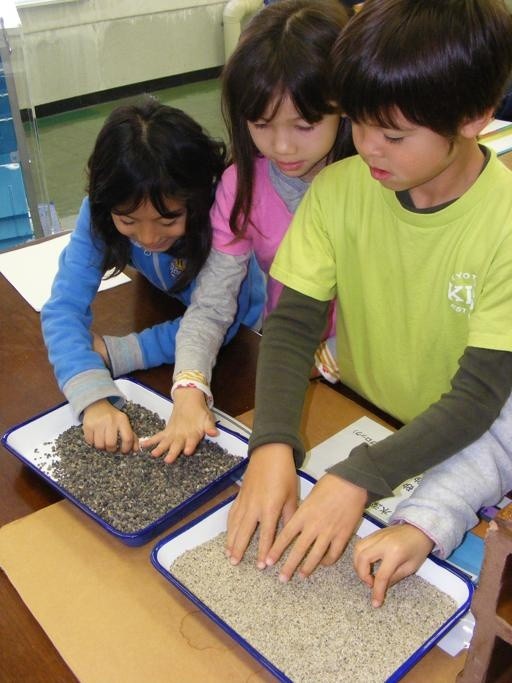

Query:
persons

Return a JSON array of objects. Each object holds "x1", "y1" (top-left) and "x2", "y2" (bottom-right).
[
  {"x1": 135, "y1": 0, "x2": 358, "y2": 463},
  {"x1": 38, "y1": 104, "x2": 267, "y2": 454},
  {"x1": 221, "y1": 0, "x2": 512, "y2": 583},
  {"x1": 351, "y1": 392, "x2": 512, "y2": 607}
]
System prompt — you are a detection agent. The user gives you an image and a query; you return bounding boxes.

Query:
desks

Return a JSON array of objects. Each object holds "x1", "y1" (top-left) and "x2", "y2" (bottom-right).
[{"x1": 0, "y1": 228, "x2": 512, "y2": 683}]
